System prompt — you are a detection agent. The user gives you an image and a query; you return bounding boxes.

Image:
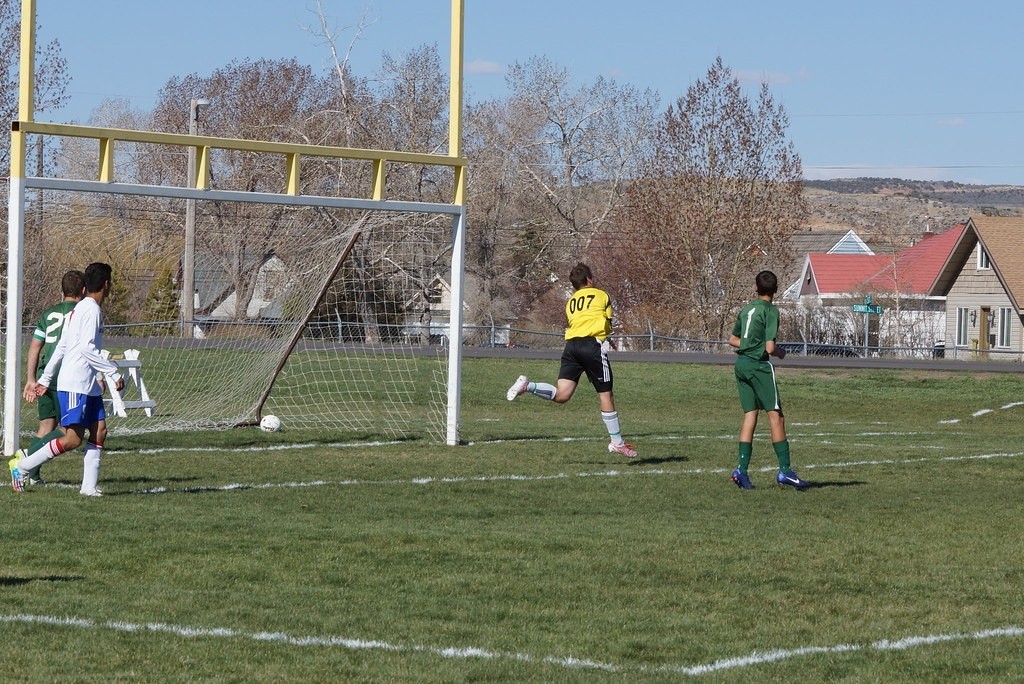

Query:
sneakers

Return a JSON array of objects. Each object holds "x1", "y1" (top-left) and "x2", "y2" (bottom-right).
[
  {"x1": 731, "y1": 469, "x2": 752, "y2": 489},
  {"x1": 506, "y1": 375, "x2": 529, "y2": 401},
  {"x1": 9, "y1": 457, "x2": 29, "y2": 491},
  {"x1": 607, "y1": 443, "x2": 637, "y2": 458},
  {"x1": 777, "y1": 470, "x2": 810, "y2": 488},
  {"x1": 14, "y1": 449, "x2": 44, "y2": 484}
]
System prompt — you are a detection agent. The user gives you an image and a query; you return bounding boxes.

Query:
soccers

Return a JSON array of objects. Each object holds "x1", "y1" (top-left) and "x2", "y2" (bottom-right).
[{"x1": 261, "y1": 415, "x2": 281, "y2": 433}]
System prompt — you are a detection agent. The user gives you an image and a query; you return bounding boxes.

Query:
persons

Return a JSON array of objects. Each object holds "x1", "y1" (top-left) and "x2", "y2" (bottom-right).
[
  {"x1": 507, "y1": 262, "x2": 638, "y2": 458},
  {"x1": 9, "y1": 262, "x2": 125, "y2": 496},
  {"x1": 15, "y1": 269, "x2": 87, "y2": 485},
  {"x1": 729, "y1": 270, "x2": 811, "y2": 490}
]
